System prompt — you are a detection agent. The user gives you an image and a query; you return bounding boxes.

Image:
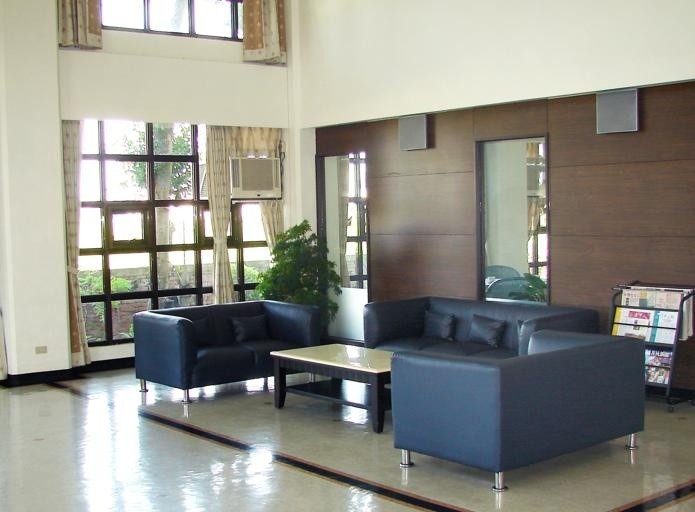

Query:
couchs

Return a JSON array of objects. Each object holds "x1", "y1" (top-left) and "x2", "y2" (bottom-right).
[
  {"x1": 132, "y1": 300, "x2": 321, "y2": 404},
  {"x1": 364, "y1": 295, "x2": 599, "y2": 409},
  {"x1": 391, "y1": 329, "x2": 645, "y2": 492}
]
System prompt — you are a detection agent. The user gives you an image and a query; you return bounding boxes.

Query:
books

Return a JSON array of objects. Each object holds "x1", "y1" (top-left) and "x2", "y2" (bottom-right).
[{"x1": 611, "y1": 285, "x2": 693, "y2": 384}]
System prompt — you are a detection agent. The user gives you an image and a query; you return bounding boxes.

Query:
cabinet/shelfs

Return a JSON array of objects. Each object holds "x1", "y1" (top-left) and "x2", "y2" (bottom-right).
[{"x1": 607, "y1": 280, "x2": 695, "y2": 413}]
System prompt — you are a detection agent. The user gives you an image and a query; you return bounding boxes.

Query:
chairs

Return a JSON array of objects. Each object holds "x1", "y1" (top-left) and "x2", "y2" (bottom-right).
[
  {"x1": 485, "y1": 277, "x2": 547, "y2": 300},
  {"x1": 484, "y1": 265, "x2": 520, "y2": 278}
]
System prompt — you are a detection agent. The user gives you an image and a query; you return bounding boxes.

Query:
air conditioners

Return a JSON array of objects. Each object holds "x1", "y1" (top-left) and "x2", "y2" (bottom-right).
[{"x1": 198, "y1": 157, "x2": 282, "y2": 201}]
